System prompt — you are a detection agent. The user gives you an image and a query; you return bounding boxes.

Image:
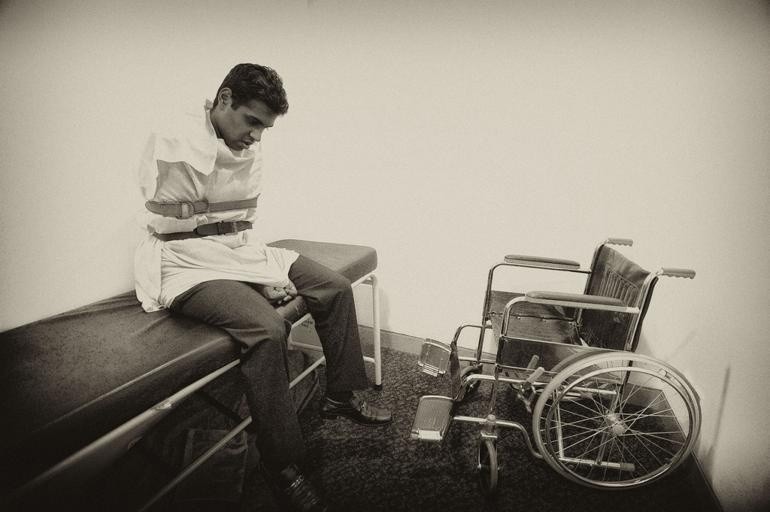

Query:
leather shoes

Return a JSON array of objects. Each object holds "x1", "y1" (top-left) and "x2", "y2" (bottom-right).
[
  {"x1": 317, "y1": 393, "x2": 393, "y2": 426},
  {"x1": 270, "y1": 475, "x2": 335, "y2": 512}
]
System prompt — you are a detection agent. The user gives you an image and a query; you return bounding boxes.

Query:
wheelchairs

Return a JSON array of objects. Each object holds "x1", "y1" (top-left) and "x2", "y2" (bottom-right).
[{"x1": 408, "y1": 237, "x2": 702, "y2": 498}]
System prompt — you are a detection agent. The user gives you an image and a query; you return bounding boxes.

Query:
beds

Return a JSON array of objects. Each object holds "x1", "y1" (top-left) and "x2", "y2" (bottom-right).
[{"x1": 0, "y1": 237, "x2": 385, "y2": 512}]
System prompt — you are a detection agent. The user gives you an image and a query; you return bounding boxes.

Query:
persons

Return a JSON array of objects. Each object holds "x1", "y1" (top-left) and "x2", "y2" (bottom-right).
[{"x1": 132, "y1": 63, "x2": 396, "y2": 512}]
